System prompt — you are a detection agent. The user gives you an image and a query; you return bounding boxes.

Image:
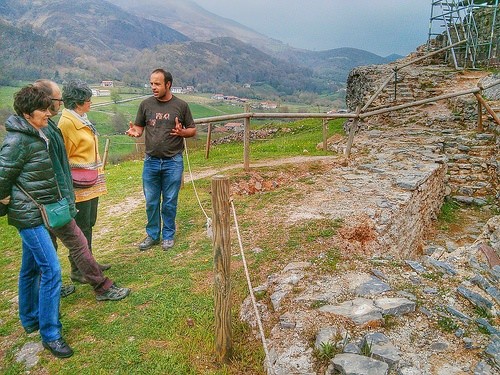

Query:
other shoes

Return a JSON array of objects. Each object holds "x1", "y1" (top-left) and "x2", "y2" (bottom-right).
[
  {"x1": 163, "y1": 239, "x2": 175, "y2": 251},
  {"x1": 70, "y1": 274, "x2": 89, "y2": 283},
  {"x1": 139, "y1": 237, "x2": 160, "y2": 250},
  {"x1": 97, "y1": 263, "x2": 110, "y2": 271},
  {"x1": 25, "y1": 312, "x2": 61, "y2": 334}
]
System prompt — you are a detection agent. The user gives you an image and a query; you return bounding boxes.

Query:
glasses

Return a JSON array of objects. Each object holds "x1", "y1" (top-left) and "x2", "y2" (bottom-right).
[
  {"x1": 81, "y1": 99, "x2": 91, "y2": 103},
  {"x1": 50, "y1": 98, "x2": 64, "y2": 103}
]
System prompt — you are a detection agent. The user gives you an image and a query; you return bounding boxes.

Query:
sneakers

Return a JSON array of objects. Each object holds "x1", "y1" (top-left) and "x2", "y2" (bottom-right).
[
  {"x1": 42, "y1": 338, "x2": 73, "y2": 358},
  {"x1": 96, "y1": 283, "x2": 130, "y2": 301},
  {"x1": 60, "y1": 283, "x2": 75, "y2": 296}
]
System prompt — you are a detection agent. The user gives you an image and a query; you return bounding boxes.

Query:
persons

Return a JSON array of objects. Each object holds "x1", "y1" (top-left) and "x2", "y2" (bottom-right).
[
  {"x1": 0, "y1": 83, "x2": 74, "y2": 358},
  {"x1": 57, "y1": 78, "x2": 112, "y2": 284},
  {"x1": 126, "y1": 68, "x2": 196, "y2": 250},
  {"x1": 32, "y1": 79, "x2": 131, "y2": 301}
]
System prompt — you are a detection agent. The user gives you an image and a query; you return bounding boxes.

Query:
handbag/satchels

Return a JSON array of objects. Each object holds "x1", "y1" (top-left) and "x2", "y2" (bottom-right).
[
  {"x1": 70, "y1": 166, "x2": 98, "y2": 188},
  {"x1": 39, "y1": 197, "x2": 72, "y2": 230}
]
